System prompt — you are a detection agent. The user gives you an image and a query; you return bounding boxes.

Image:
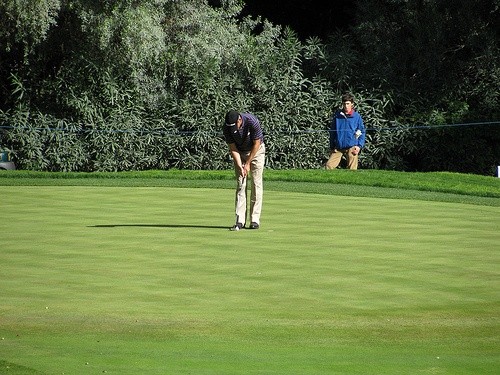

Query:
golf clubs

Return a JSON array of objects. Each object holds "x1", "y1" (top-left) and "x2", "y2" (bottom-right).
[{"x1": 229, "y1": 174, "x2": 245, "y2": 232}]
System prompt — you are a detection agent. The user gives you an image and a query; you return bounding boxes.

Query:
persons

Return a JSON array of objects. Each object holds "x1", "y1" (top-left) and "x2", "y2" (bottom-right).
[
  {"x1": 221, "y1": 109, "x2": 266, "y2": 229},
  {"x1": 324, "y1": 95, "x2": 367, "y2": 170}
]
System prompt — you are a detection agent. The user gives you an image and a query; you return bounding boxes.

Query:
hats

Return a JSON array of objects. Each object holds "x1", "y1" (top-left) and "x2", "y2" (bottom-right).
[{"x1": 223, "y1": 111, "x2": 241, "y2": 135}]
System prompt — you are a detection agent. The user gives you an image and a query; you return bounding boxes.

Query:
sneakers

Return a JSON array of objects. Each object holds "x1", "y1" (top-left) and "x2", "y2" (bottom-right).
[
  {"x1": 233, "y1": 222, "x2": 245, "y2": 230},
  {"x1": 249, "y1": 221, "x2": 260, "y2": 229}
]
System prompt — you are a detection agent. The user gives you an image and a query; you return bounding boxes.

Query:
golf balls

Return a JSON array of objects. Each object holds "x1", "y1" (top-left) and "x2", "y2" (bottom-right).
[{"x1": 234, "y1": 225, "x2": 239, "y2": 231}]
